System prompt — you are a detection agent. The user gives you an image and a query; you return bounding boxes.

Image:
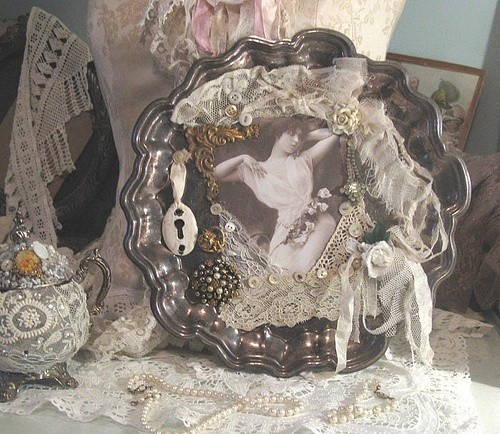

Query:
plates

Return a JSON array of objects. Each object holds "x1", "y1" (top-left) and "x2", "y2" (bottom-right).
[{"x1": 122, "y1": 23, "x2": 471, "y2": 375}]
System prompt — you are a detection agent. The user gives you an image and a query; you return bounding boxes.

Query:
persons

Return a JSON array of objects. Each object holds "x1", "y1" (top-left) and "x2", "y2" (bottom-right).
[{"x1": 212, "y1": 114, "x2": 340, "y2": 274}]
